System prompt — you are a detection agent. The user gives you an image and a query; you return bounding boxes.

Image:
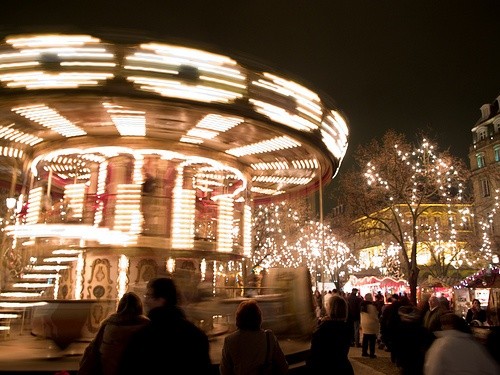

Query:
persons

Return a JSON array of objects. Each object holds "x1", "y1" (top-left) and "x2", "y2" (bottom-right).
[
  {"x1": 375, "y1": 291, "x2": 384, "y2": 318},
  {"x1": 431, "y1": 293, "x2": 449, "y2": 309},
  {"x1": 117, "y1": 278, "x2": 211, "y2": 375},
  {"x1": 381, "y1": 294, "x2": 437, "y2": 375},
  {"x1": 85, "y1": 292, "x2": 147, "y2": 375},
  {"x1": 423, "y1": 313, "x2": 500, "y2": 375},
  {"x1": 359, "y1": 293, "x2": 380, "y2": 358},
  {"x1": 466, "y1": 299, "x2": 487, "y2": 327},
  {"x1": 314, "y1": 289, "x2": 351, "y2": 309},
  {"x1": 485, "y1": 309, "x2": 500, "y2": 367},
  {"x1": 348, "y1": 288, "x2": 364, "y2": 347},
  {"x1": 220, "y1": 300, "x2": 288, "y2": 375},
  {"x1": 306, "y1": 296, "x2": 354, "y2": 375}
]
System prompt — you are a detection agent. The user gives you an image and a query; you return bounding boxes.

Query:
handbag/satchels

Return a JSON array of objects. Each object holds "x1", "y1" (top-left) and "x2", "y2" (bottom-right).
[{"x1": 77, "y1": 346, "x2": 101, "y2": 375}]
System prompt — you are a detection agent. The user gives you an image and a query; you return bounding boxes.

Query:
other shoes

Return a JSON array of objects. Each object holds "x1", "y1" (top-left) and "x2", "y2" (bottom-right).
[{"x1": 362, "y1": 353, "x2": 376, "y2": 358}]
